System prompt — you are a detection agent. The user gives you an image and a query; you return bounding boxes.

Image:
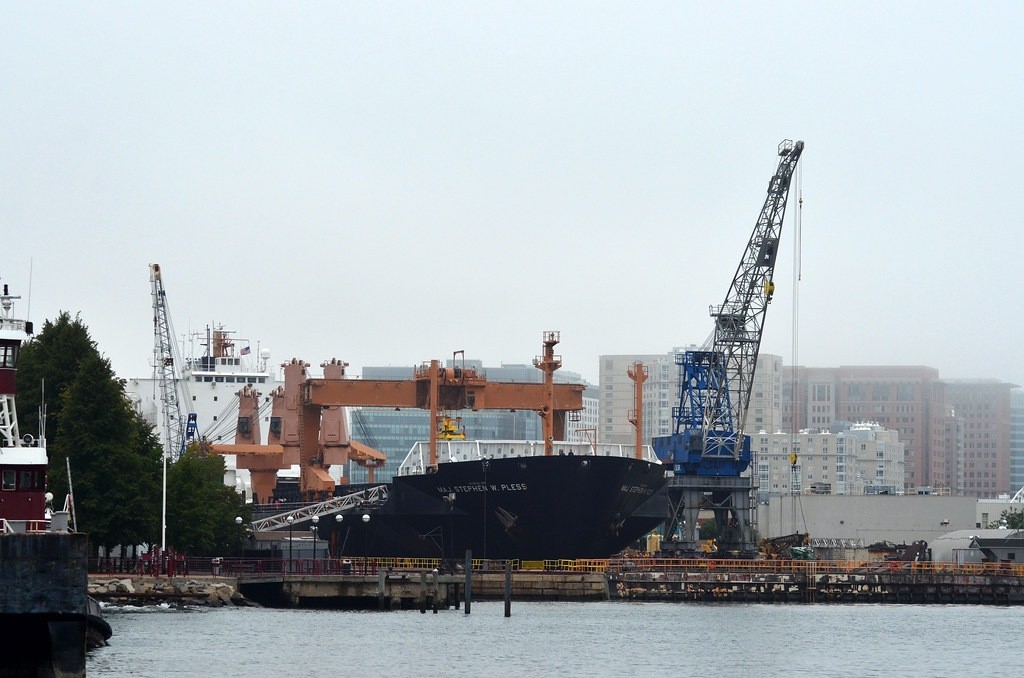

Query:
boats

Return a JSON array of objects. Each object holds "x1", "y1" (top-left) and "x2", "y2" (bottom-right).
[{"x1": 317, "y1": 456, "x2": 682, "y2": 561}]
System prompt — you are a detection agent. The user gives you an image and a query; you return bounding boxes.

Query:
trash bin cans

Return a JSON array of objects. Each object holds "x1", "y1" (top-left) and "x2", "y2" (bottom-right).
[
  {"x1": 212, "y1": 557, "x2": 223, "y2": 575},
  {"x1": 340, "y1": 558, "x2": 352, "y2": 573}
]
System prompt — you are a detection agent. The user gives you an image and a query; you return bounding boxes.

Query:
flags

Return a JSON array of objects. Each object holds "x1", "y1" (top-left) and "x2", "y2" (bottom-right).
[{"x1": 241, "y1": 346, "x2": 250, "y2": 355}]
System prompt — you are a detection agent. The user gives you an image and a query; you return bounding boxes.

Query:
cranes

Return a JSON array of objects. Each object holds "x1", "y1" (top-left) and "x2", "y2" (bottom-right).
[
  {"x1": 147, "y1": 263, "x2": 206, "y2": 466},
  {"x1": 650, "y1": 137, "x2": 806, "y2": 561}
]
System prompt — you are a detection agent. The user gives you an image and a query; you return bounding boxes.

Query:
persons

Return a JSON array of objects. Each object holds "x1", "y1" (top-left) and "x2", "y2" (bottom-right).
[{"x1": 442, "y1": 417, "x2": 464, "y2": 435}]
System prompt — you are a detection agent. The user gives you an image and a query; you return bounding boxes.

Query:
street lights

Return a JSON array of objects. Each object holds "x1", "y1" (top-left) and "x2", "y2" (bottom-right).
[
  {"x1": 235, "y1": 517, "x2": 243, "y2": 564},
  {"x1": 287, "y1": 515, "x2": 294, "y2": 572},
  {"x1": 335, "y1": 514, "x2": 343, "y2": 560},
  {"x1": 361, "y1": 514, "x2": 371, "y2": 575},
  {"x1": 311, "y1": 516, "x2": 319, "y2": 573}
]
